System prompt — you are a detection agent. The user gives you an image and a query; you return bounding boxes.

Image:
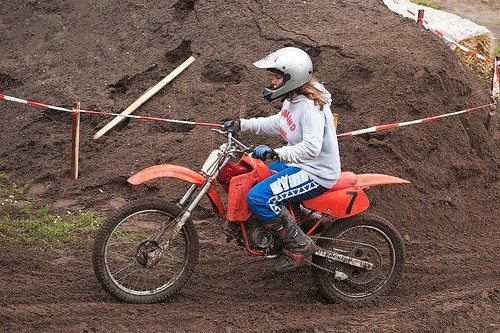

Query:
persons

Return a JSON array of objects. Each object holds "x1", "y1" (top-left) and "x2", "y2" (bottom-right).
[{"x1": 222, "y1": 47, "x2": 340, "y2": 273}]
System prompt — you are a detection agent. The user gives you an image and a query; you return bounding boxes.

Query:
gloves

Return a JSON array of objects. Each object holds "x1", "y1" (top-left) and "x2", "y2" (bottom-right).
[
  {"x1": 252, "y1": 145, "x2": 278, "y2": 162},
  {"x1": 222, "y1": 119, "x2": 239, "y2": 138}
]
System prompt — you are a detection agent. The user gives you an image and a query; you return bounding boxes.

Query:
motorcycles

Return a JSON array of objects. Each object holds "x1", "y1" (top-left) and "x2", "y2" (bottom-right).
[{"x1": 93, "y1": 123, "x2": 411, "y2": 305}]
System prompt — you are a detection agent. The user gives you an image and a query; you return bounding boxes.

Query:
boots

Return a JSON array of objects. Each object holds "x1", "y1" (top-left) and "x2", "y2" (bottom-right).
[{"x1": 262, "y1": 205, "x2": 318, "y2": 273}]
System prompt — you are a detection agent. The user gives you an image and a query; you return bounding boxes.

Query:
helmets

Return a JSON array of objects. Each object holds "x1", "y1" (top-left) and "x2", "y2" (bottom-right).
[{"x1": 252, "y1": 46, "x2": 313, "y2": 101}]
